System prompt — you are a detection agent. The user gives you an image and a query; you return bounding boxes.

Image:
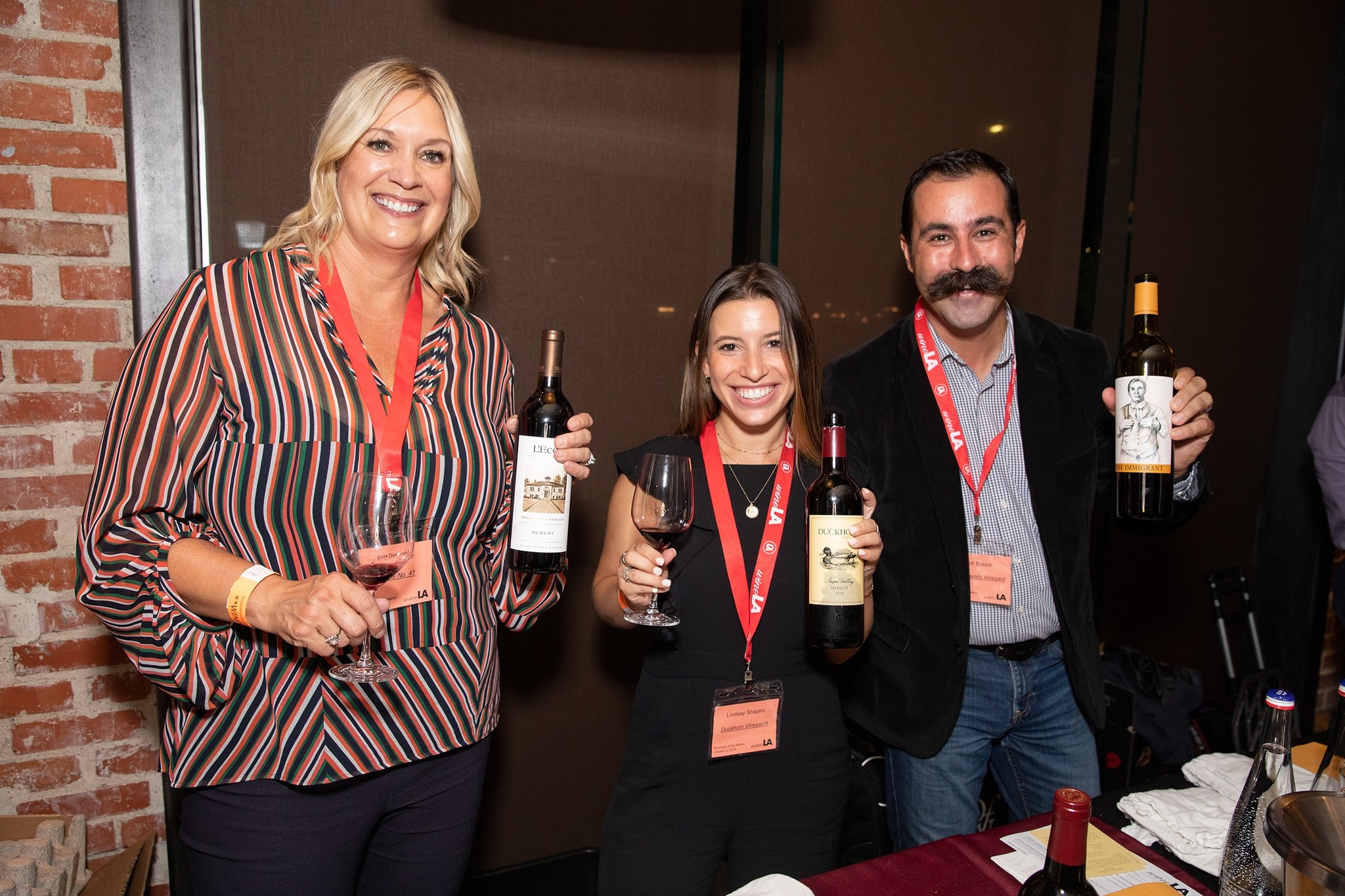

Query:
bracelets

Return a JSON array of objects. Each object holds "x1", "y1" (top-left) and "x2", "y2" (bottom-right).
[
  {"x1": 618, "y1": 587, "x2": 636, "y2": 613},
  {"x1": 864, "y1": 584, "x2": 874, "y2": 598},
  {"x1": 226, "y1": 564, "x2": 283, "y2": 629}
]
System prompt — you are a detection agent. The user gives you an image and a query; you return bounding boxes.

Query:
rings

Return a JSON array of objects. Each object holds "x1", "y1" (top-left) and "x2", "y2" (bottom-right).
[
  {"x1": 325, "y1": 627, "x2": 342, "y2": 646},
  {"x1": 621, "y1": 551, "x2": 633, "y2": 568},
  {"x1": 623, "y1": 566, "x2": 633, "y2": 582},
  {"x1": 1199, "y1": 405, "x2": 1213, "y2": 413},
  {"x1": 578, "y1": 452, "x2": 596, "y2": 466},
  {"x1": 876, "y1": 523, "x2": 879, "y2": 533}
]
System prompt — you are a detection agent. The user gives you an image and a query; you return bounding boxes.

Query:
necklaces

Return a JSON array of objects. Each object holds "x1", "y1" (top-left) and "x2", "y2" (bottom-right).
[
  {"x1": 715, "y1": 422, "x2": 784, "y2": 455},
  {"x1": 716, "y1": 441, "x2": 781, "y2": 518}
]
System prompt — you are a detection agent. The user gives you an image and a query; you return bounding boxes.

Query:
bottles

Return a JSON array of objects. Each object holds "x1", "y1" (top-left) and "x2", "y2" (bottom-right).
[
  {"x1": 507, "y1": 328, "x2": 575, "y2": 573},
  {"x1": 1017, "y1": 787, "x2": 1099, "y2": 896},
  {"x1": 1115, "y1": 272, "x2": 1177, "y2": 523},
  {"x1": 803, "y1": 411, "x2": 866, "y2": 649},
  {"x1": 1309, "y1": 680, "x2": 1345, "y2": 790},
  {"x1": 1220, "y1": 689, "x2": 1298, "y2": 896}
]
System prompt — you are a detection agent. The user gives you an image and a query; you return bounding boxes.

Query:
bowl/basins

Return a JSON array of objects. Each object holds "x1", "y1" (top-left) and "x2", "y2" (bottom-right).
[{"x1": 1262, "y1": 791, "x2": 1345, "y2": 896}]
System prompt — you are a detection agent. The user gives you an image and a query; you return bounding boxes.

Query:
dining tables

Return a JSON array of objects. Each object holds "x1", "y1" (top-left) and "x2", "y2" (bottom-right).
[{"x1": 801, "y1": 737, "x2": 1344, "y2": 896}]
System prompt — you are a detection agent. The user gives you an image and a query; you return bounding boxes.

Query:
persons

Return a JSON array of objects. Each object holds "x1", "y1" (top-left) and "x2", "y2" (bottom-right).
[
  {"x1": 1305, "y1": 372, "x2": 1345, "y2": 630},
  {"x1": 817, "y1": 148, "x2": 1215, "y2": 854},
  {"x1": 75, "y1": 58, "x2": 595, "y2": 896},
  {"x1": 588, "y1": 261, "x2": 884, "y2": 896}
]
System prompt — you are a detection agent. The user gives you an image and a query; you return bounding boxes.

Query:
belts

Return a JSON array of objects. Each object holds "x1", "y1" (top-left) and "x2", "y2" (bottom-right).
[{"x1": 969, "y1": 631, "x2": 1061, "y2": 661}]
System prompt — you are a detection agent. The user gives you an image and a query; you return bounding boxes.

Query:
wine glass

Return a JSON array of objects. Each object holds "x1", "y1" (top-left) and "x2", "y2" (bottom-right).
[
  {"x1": 328, "y1": 473, "x2": 416, "y2": 682},
  {"x1": 623, "y1": 452, "x2": 695, "y2": 626}
]
